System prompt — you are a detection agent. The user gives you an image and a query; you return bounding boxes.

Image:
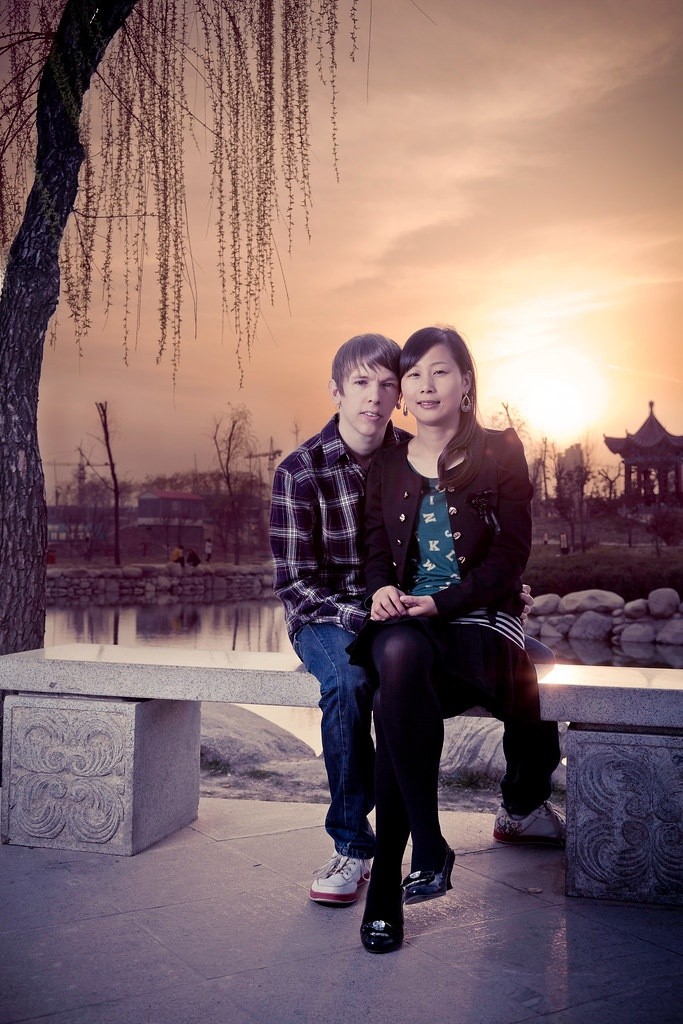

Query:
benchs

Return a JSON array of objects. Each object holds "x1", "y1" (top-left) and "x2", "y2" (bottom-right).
[{"x1": 0, "y1": 644, "x2": 682, "y2": 905}]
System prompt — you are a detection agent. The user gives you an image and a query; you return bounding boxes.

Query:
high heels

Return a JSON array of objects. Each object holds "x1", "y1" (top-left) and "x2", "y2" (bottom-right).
[
  {"x1": 361, "y1": 879, "x2": 404, "y2": 954},
  {"x1": 401, "y1": 837, "x2": 454, "y2": 906}
]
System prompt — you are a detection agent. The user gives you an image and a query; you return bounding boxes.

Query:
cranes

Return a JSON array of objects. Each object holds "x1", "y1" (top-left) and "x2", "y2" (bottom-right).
[
  {"x1": 42, "y1": 454, "x2": 112, "y2": 506},
  {"x1": 243, "y1": 436, "x2": 283, "y2": 485}
]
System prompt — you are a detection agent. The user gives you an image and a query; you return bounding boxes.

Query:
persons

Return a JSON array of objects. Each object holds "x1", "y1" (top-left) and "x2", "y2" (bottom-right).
[
  {"x1": 186, "y1": 547, "x2": 199, "y2": 563},
  {"x1": 266, "y1": 333, "x2": 568, "y2": 906},
  {"x1": 171, "y1": 545, "x2": 185, "y2": 568},
  {"x1": 346, "y1": 326, "x2": 537, "y2": 955},
  {"x1": 204, "y1": 538, "x2": 213, "y2": 562}
]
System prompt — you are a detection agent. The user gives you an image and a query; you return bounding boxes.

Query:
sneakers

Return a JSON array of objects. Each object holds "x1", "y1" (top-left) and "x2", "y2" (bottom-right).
[
  {"x1": 492, "y1": 799, "x2": 566, "y2": 845},
  {"x1": 309, "y1": 849, "x2": 370, "y2": 905}
]
